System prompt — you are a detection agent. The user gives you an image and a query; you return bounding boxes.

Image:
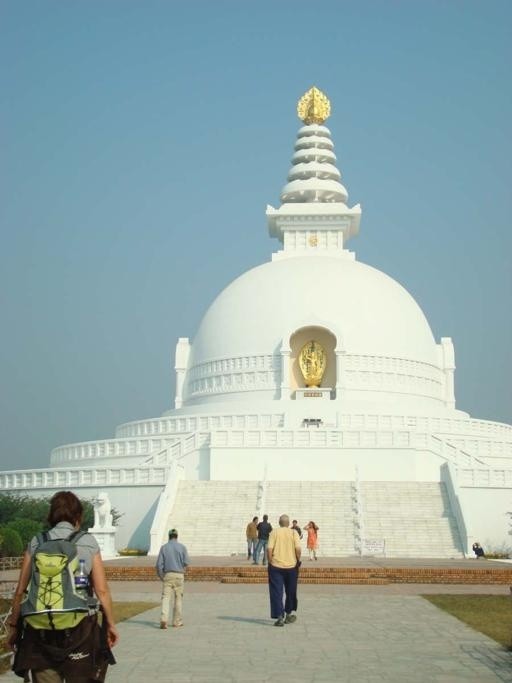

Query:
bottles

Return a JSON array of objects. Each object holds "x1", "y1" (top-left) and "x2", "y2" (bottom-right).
[{"x1": 73, "y1": 559, "x2": 88, "y2": 598}]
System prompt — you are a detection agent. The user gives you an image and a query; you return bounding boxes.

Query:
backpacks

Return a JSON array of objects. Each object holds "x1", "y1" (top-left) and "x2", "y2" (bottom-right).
[{"x1": 22, "y1": 530, "x2": 100, "y2": 647}]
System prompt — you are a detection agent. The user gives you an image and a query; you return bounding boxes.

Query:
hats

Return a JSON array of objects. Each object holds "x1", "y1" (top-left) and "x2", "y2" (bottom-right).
[{"x1": 167, "y1": 529, "x2": 178, "y2": 536}]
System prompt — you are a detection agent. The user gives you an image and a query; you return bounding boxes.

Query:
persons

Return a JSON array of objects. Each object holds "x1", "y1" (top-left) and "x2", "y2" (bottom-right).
[
  {"x1": 6, "y1": 489, "x2": 118, "y2": 683},
  {"x1": 244, "y1": 513, "x2": 320, "y2": 565},
  {"x1": 155, "y1": 527, "x2": 190, "y2": 632},
  {"x1": 265, "y1": 514, "x2": 302, "y2": 626}
]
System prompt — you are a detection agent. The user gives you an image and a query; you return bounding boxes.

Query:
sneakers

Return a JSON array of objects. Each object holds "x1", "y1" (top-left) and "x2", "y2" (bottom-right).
[
  {"x1": 285, "y1": 615, "x2": 295, "y2": 624},
  {"x1": 172, "y1": 621, "x2": 182, "y2": 627},
  {"x1": 161, "y1": 618, "x2": 167, "y2": 630},
  {"x1": 275, "y1": 620, "x2": 284, "y2": 626}
]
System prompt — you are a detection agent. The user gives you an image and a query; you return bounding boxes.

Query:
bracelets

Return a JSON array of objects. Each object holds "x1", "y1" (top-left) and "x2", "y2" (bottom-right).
[{"x1": 10, "y1": 623, "x2": 17, "y2": 628}]
